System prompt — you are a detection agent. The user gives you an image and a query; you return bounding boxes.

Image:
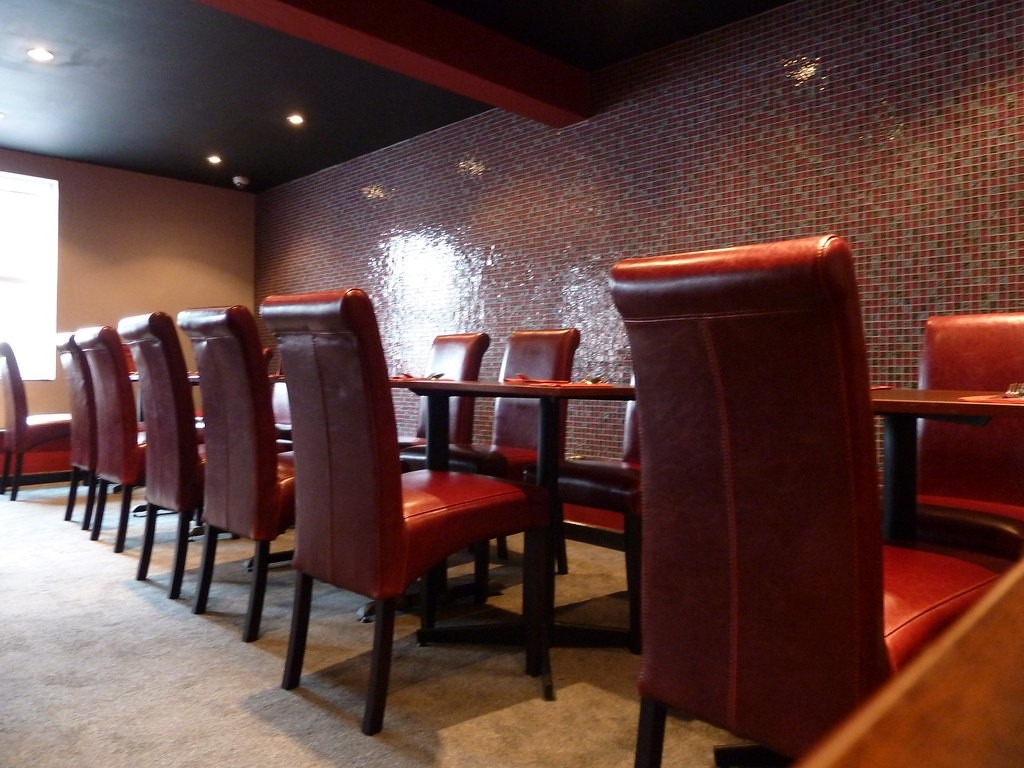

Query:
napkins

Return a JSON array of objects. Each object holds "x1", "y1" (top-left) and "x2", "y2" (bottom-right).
[
  {"x1": 504, "y1": 377, "x2": 571, "y2": 384},
  {"x1": 527, "y1": 382, "x2": 613, "y2": 387},
  {"x1": 957, "y1": 395, "x2": 1024, "y2": 405},
  {"x1": 870, "y1": 385, "x2": 894, "y2": 390}
]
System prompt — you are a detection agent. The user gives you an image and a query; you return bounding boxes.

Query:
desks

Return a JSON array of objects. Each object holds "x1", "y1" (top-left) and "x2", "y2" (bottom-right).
[
  {"x1": 711, "y1": 389, "x2": 1024, "y2": 768},
  {"x1": 389, "y1": 378, "x2": 641, "y2": 700},
  {"x1": 114, "y1": 373, "x2": 204, "y2": 517},
  {"x1": 246, "y1": 373, "x2": 296, "y2": 569}
]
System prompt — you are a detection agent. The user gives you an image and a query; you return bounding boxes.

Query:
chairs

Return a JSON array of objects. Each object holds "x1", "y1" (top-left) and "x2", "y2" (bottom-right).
[{"x1": 0, "y1": 235, "x2": 1024, "y2": 768}]
